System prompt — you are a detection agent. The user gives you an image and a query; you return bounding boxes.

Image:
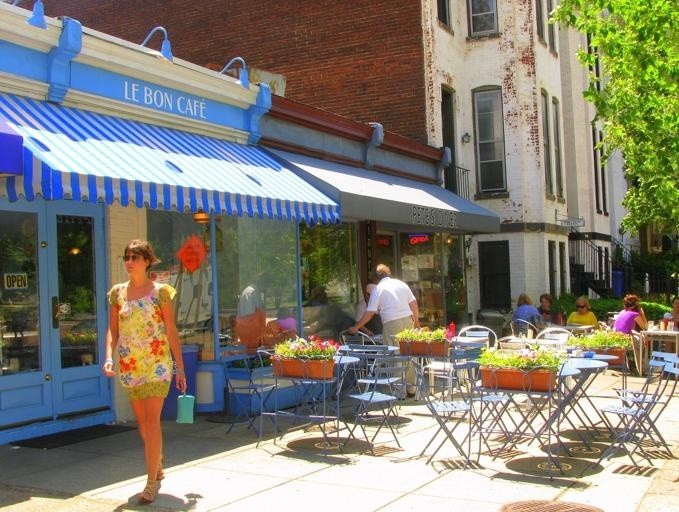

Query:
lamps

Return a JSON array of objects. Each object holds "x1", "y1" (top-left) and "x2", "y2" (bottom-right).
[
  {"x1": 11, "y1": 0, "x2": 48, "y2": 30},
  {"x1": 140, "y1": 23, "x2": 172, "y2": 65},
  {"x1": 218, "y1": 55, "x2": 250, "y2": 90},
  {"x1": 461, "y1": 131, "x2": 471, "y2": 143},
  {"x1": 466, "y1": 237, "x2": 473, "y2": 253},
  {"x1": 193, "y1": 210, "x2": 221, "y2": 227}
]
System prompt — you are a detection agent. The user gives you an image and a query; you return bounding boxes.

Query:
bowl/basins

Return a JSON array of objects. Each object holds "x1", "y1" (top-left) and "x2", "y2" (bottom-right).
[
  {"x1": 572, "y1": 350, "x2": 582, "y2": 356},
  {"x1": 584, "y1": 352, "x2": 595, "y2": 358}
]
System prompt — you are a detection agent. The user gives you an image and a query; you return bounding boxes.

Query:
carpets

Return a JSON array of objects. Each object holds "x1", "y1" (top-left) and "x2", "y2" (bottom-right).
[{"x1": 8, "y1": 422, "x2": 138, "y2": 450}]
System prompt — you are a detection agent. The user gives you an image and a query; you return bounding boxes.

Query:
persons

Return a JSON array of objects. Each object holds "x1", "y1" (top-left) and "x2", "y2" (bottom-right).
[
  {"x1": 349, "y1": 263, "x2": 419, "y2": 400},
  {"x1": 614, "y1": 294, "x2": 649, "y2": 375},
  {"x1": 536, "y1": 294, "x2": 556, "y2": 324},
  {"x1": 190, "y1": 258, "x2": 213, "y2": 319},
  {"x1": 229, "y1": 270, "x2": 346, "y2": 340},
  {"x1": 665, "y1": 296, "x2": 679, "y2": 353},
  {"x1": 354, "y1": 284, "x2": 386, "y2": 377},
  {"x1": 566, "y1": 296, "x2": 598, "y2": 334},
  {"x1": 512, "y1": 293, "x2": 546, "y2": 339},
  {"x1": 103, "y1": 238, "x2": 187, "y2": 503}
]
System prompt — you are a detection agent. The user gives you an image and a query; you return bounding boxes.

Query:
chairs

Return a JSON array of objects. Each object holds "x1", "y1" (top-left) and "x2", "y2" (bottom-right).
[{"x1": 217, "y1": 317, "x2": 679, "y2": 471}]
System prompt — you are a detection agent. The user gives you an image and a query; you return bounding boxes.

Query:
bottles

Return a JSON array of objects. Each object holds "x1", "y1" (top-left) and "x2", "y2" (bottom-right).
[
  {"x1": 519, "y1": 329, "x2": 533, "y2": 341},
  {"x1": 450, "y1": 320, "x2": 455, "y2": 336}
]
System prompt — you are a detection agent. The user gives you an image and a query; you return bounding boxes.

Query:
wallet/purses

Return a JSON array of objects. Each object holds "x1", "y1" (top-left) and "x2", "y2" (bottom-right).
[{"x1": 177, "y1": 395, "x2": 195, "y2": 424}]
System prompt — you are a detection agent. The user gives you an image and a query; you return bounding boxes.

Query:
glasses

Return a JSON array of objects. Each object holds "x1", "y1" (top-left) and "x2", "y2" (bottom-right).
[
  {"x1": 576, "y1": 304, "x2": 586, "y2": 309},
  {"x1": 123, "y1": 255, "x2": 141, "y2": 261}
]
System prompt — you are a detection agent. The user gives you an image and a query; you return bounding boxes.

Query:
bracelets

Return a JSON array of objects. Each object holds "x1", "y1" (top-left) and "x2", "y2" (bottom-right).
[{"x1": 105, "y1": 359, "x2": 114, "y2": 362}]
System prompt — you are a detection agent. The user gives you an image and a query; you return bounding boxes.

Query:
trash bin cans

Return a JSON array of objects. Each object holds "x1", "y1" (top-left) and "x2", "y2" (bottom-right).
[
  {"x1": 160, "y1": 345, "x2": 199, "y2": 421},
  {"x1": 612, "y1": 271, "x2": 623, "y2": 297}
]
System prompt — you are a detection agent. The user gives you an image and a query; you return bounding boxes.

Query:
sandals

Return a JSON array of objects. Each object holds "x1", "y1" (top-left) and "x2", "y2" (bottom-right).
[{"x1": 139, "y1": 479, "x2": 160, "y2": 502}]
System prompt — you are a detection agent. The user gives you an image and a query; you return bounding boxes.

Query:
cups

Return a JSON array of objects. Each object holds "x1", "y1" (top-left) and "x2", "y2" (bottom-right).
[
  {"x1": 659, "y1": 322, "x2": 665, "y2": 331},
  {"x1": 654, "y1": 327, "x2": 659, "y2": 330},
  {"x1": 668, "y1": 321, "x2": 674, "y2": 331},
  {"x1": 648, "y1": 321, "x2": 654, "y2": 330}
]
